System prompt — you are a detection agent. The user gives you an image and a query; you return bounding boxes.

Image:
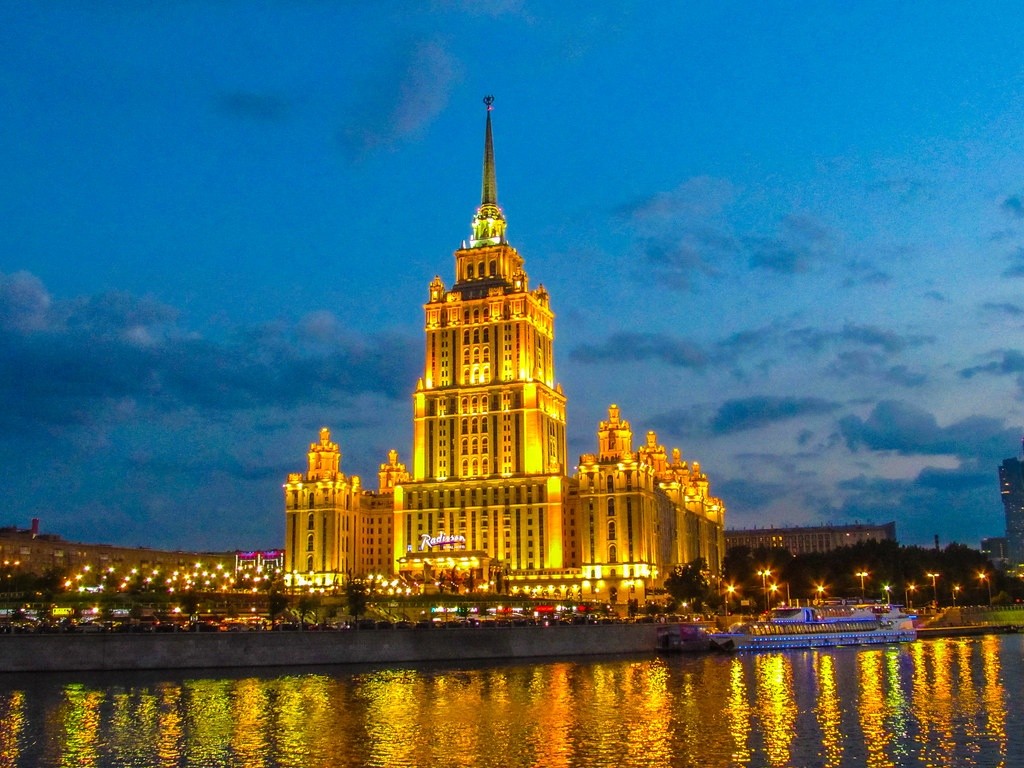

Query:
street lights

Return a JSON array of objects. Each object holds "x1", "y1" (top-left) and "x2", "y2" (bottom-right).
[
  {"x1": 979, "y1": 573, "x2": 992, "y2": 607},
  {"x1": 952, "y1": 586, "x2": 960, "y2": 607},
  {"x1": 766, "y1": 585, "x2": 776, "y2": 612},
  {"x1": 762, "y1": 570, "x2": 771, "y2": 611},
  {"x1": 905, "y1": 585, "x2": 915, "y2": 610},
  {"x1": 855, "y1": 572, "x2": 868, "y2": 600},
  {"x1": 927, "y1": 573, "x2": 939, "y2": 599},
  {"x1": 725, "y1": 585, "x2": 735, "y2": 627}
]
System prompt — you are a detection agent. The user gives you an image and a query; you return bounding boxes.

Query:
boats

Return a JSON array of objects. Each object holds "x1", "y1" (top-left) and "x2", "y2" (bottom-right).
[{"x1": 708, "y1": 605, "x2": 919, "y2": 654}]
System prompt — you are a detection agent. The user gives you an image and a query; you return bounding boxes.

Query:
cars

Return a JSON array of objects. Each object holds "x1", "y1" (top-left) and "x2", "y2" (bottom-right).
[{"x1": 0, "y1": 604, "x2": 769, "y2": 639}]
